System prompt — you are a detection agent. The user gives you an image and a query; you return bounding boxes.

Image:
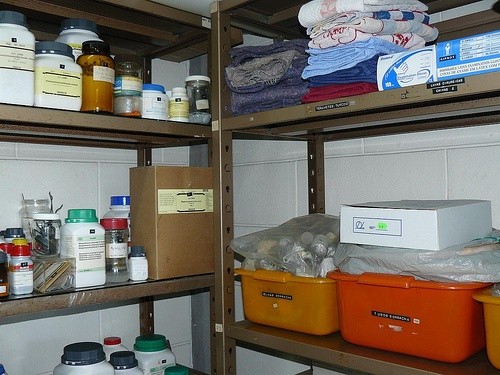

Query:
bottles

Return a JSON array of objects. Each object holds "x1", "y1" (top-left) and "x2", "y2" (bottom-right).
[
  {"x1": 0, "y1": 194, "x2": 149, "y2": 299},
  {"x1": 0, "y1": 333, "x2": 189, "y2": 375},
  {"x1": 0, "y1": 11, "x2": 211, "y2": 124}
]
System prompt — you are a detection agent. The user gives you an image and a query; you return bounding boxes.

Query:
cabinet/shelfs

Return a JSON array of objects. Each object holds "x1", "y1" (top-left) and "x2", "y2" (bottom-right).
[
  {"x1": 210, "y1": 0, "x2": 500, "y2": 375},
  {"x1": 0, "y1": 0, "x2": 231, "y2": 375}
]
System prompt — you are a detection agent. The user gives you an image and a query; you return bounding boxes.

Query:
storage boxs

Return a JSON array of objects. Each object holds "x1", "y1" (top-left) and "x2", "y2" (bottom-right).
[
  {"x1": 328, "y1": 270, "x2": 493, "y2": 362},
  {"x1": 377, "y1": 28, "x2": 500, "y2": 91},
  {"x1": 470, "y1": 287, "x2": 500, "y2": 370},
  {"x1": 129, "y1": 165, "x2": 214, "y2": 279},
  {"x1": 340, "y1": 197, "x2": 492, "y2": 251},
  {"x1": 31, "y1": 255, "x2": 76, "y2": 294},
  {"x1": 234, "y1": 262, "x2": 339, "y2": 335}
]
